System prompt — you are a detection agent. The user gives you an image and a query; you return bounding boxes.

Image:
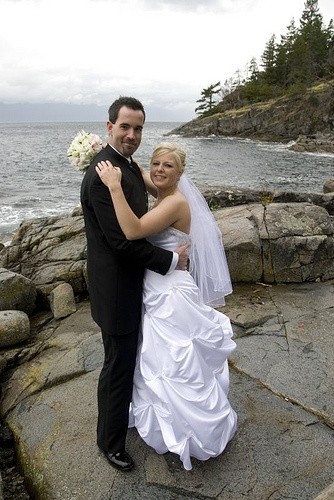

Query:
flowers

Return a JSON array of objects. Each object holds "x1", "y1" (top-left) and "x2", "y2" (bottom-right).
[{"x1": 67, "y1": 130, "x2": 104, "y2": 173}]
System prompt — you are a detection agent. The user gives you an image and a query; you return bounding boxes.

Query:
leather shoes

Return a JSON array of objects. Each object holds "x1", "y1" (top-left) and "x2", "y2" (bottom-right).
[{"x1": 103, "y1": 448, "x2": 134, "y2": 470}]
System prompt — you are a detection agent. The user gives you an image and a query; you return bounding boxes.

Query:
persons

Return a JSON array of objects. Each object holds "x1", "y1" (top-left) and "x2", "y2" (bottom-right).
[
  {"x1": 133, "y1": 142, "x2": 238, "y2": 470},
  {"x1": 79, "y1": 97, "x2": 192, "y2": 471}
]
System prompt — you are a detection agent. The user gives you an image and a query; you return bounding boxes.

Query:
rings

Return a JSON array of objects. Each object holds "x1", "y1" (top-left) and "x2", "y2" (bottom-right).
[{"x1": 100, "y1": 167, "x2": 103, "y2": 169}]
